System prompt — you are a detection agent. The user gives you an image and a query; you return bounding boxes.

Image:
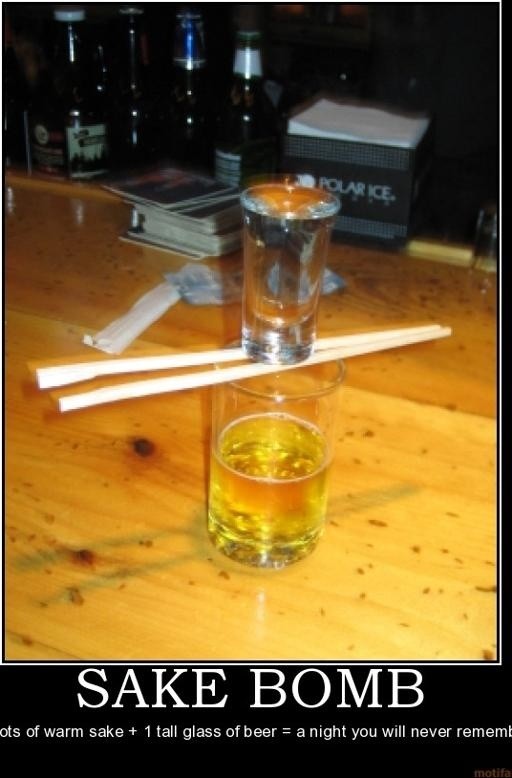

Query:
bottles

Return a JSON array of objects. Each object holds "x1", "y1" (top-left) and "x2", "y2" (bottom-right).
[
  {"x1": 214, "y1": 29, "x2": 281, "y2": 187},
  {"x1": 29, "y1": 7, "x2": 114, "y2": 182},
  {"x1": 111, "y1": 7, "x2": 165, "y2": 155},
  {"x1": 163, "y1": 7, "x2": 221, "y2": 163}
]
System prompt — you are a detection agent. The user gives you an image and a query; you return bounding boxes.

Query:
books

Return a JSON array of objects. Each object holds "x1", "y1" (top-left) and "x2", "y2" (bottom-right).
[{"x1": 101, "y1": 163, "x2": 244, "y2": 259}]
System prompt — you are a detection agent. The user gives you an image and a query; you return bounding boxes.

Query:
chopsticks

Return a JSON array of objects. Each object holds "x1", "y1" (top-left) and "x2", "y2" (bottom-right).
[{"x1": 29, "y1": 323, "x2": 453, "y2": 415}]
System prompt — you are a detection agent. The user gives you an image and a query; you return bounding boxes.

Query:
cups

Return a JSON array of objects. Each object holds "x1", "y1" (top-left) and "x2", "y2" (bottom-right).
[
  {"x1": 207, "y1": 338, "x2": 346, "y2": 569},
  {"x1": 238, "y1": 184, "x2": 341, "y2": 364},
  {"x1": 470, "y1": 202, "x2": 496, "y2": 293}
]
